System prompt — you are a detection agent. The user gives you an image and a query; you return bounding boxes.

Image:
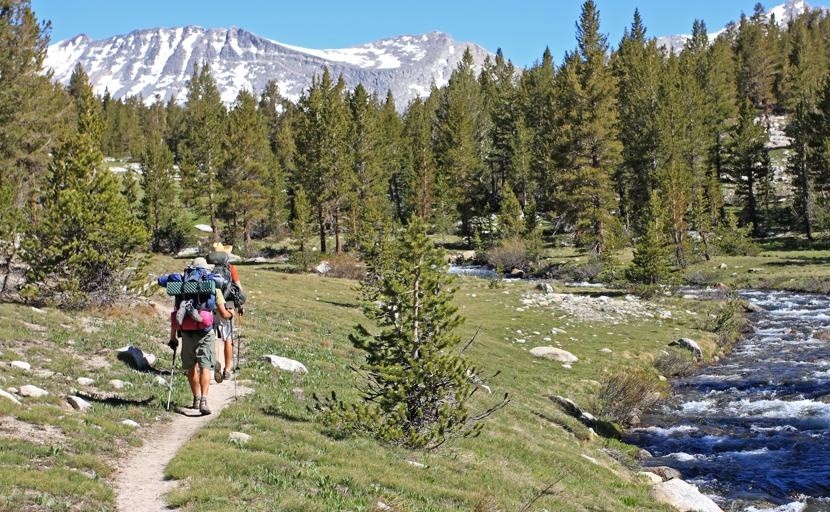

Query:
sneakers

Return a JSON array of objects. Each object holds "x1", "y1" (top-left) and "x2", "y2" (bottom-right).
[
  {"x1": 215, "y1": 361, "x2": 229, "y2": 383},
  {"x1": 193, "y1": 399, "x2": 211, "y2": 414}
]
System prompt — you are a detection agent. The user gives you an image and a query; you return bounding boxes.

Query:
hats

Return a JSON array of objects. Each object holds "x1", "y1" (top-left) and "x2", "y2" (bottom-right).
[
  {"x1": 192, "y1": 257, "x2": 215, "y2": 269},
  {"x1": 212, "y1": 242, "x2": 232, "y2": 254}
]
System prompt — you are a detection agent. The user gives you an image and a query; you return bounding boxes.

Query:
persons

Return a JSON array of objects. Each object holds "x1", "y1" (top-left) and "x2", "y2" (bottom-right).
[
  {"x1": 204, "y1": 241, "x2": 246, "y2": 383},
  {"x1": 168, "y1": 256, "x2": 236, "y2": 414}
]
